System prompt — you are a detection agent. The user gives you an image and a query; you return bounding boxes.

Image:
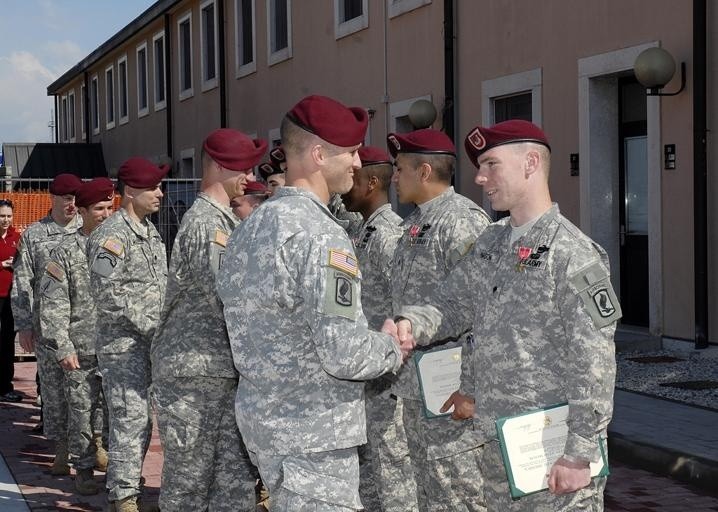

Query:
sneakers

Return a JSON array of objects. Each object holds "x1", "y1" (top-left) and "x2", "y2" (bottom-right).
[{"x1": 0, "y1": 391, "x2": 22, "y2": 402}]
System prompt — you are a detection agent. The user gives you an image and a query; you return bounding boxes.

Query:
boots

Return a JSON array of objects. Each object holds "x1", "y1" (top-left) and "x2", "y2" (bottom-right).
[
  {"x1": 52, "y1": 462, "x2": 70, "y2": 475},
  {"x1": 75, "y1": 468, "x2": 98, "y2": 495},
  {"x1": 95, "y1": 435, "x2": 109, "y2": 472},
  {"x1": 113, "y1": 494, "x2": 144, "y2": 512},
  {"x1": 255, "y1": 480, "x2": 270, "y2": 512}
]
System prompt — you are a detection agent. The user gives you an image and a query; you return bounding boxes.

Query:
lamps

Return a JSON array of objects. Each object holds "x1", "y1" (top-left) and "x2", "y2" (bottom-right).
[
  {"x1": 408, "y1": 98, "x2": 448, "y2": 132},
  {"x1": 632, "y1": 46, "x2": 689, "y2": 97}
]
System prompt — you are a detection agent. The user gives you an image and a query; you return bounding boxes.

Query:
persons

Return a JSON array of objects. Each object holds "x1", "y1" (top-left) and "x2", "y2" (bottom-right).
[
  {"x1": 1, "y1": 159, "x2": 169, "y2": 512},
  {"x1": 220, "y1": 96, "x2": 623, "y2": 512},
  {"x1": 151, "y1": 129, "x2": 270, "y2": 512}
]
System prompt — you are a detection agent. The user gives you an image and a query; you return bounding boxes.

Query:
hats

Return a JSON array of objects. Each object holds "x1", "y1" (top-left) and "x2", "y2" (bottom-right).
[
  {"x1": 286, "y1": 96, "x2": 368, "y2": 146},
  {"x1": 464, "y1": 119, "x2": 551, "y2": 169},
  {"x1": 118, "y1": 158, "x2": 171, "y2": 188},
  {"x1": 358, "y1": 146, "x2": 393, "y2": 166},
  {"x1": 75, "y1": 177, "x2": 115, "y2": 207},
  {"x1": 203, "y1": 128, "x2": 285, "y2": 194},
  {"x1": 387, "y1": 129, "x2": 457, "y2": 158},
  {"x1": 49, "y1": 174, "x2": 81, "y2": 194}
]
